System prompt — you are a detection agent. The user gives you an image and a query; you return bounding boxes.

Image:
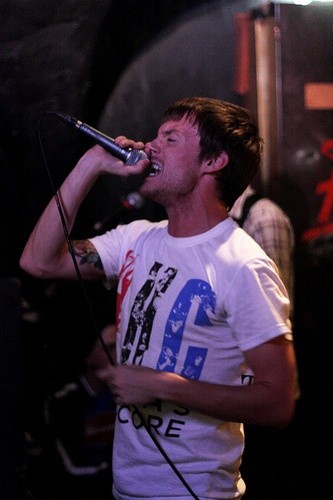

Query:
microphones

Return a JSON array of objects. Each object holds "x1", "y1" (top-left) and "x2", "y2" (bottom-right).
[{"x1": 54, "y1": 111, "x2": 149, "y2": 171}]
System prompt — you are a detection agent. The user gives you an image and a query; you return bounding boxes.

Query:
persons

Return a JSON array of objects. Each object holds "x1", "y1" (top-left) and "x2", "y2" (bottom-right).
[
  {"x1": 229, "y1": 180, "x2": 296, "y2": 304},
  {"x1": 19, "y1": 95, "x2": 297, "y2": 500},
  {"x1": 92, "y1": 324, "x2": 118, "y2": 373}
]
